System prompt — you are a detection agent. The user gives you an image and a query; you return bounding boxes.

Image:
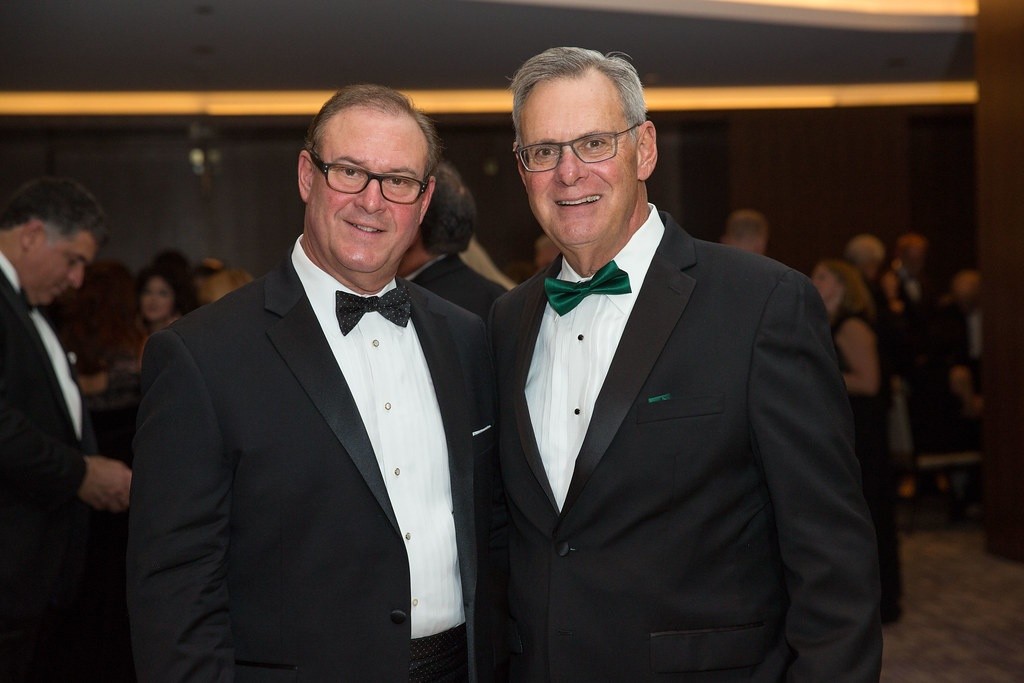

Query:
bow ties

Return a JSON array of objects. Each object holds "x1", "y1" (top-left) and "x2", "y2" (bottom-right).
[
  {"x1": 336, "y1": 286, "x2": 411, "y2": 336},
  {"x1": 544, "y1": 260, "x2": 632, "y2": 317}
]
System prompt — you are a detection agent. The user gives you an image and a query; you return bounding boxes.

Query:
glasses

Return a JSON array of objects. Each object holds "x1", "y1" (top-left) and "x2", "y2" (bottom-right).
[
  {"x1": 309, "y1": 149, "x2": 430, "y2": 205},
  {"x1": 512, "y1": 120, "x2": 643, "y2": 172}
]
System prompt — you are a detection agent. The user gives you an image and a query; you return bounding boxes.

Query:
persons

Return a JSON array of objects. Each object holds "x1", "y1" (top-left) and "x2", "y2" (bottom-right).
[
  {"x1": 808, "y1": 257, "x2": 904, "y2": 627},
  {"x1": 0, "y1": 174, "x2": 132, "y2": 681},
  {"x1": 718, "y1": 208, "x2": 768, "y2": 255},
  {"x1": 124, "y1": 82, "x2": 511, "y2": 682},
  {"x1": 842, "y1": 231, "x2": 981, "y2": 451},
  {"x1": 495, "y1": 234, "x2": 557, "y2": 280},
  {"x1": 78, "y1": 247, "x2": 201, "y2": 393},
  {"x1": 489, "y1": 43, "x2": 883, "y2": 683},
  {"x1": 397, "y1": 161, "x2": 510, "y2": 318}
]
